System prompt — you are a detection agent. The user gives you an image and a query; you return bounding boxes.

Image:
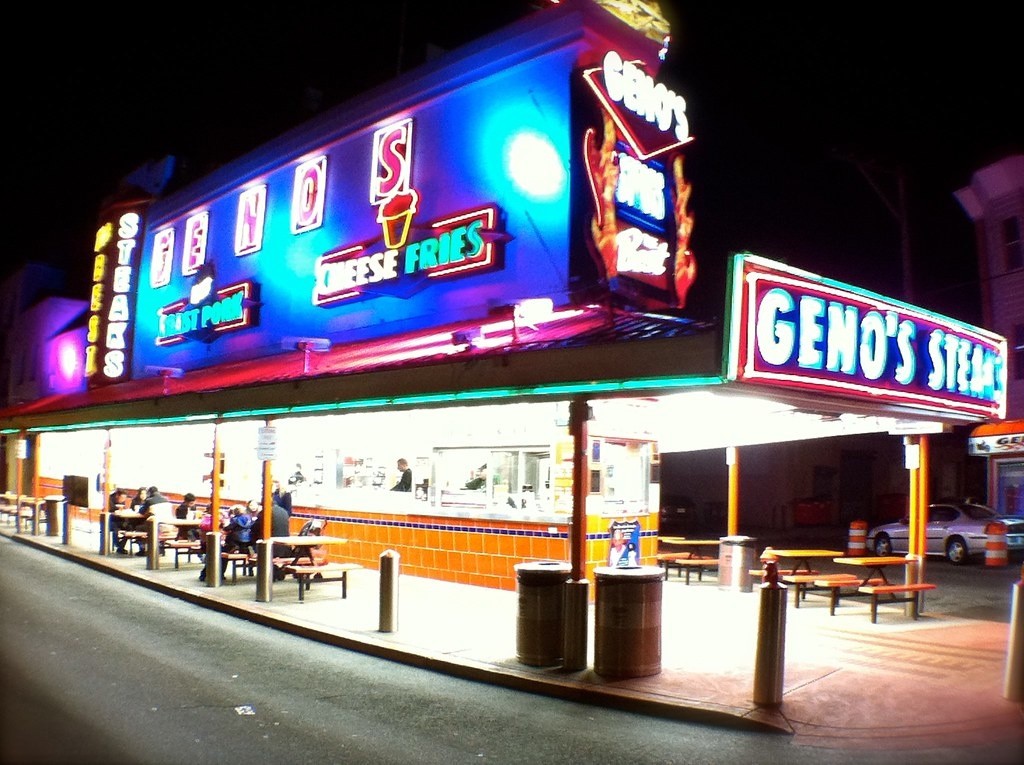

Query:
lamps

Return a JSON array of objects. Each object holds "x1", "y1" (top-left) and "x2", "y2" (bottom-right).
[
  {"x1": 450, "y1": 326, "x2": 481, "y2": 352},
  {"x1": 144, "y1": 365, "x2": 186, "y2": 394},
  {"x1": 509, "y1": 296, "x2": 552, "y2": 342},
  {"x1": 281, "y1": 335, "x2": 333, "y2": 374}
]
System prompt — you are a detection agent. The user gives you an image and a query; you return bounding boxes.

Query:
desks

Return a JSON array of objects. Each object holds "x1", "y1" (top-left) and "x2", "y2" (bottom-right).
[
  {"x1": 661, "y1": 539, "x2": 722, "y2": 585},
  {"x1": 101, "y1": 512, "x2": 143, "y2": 555},
  {"x1": 159, "y1": 518, "x2": 207, "y2": 567},
  {"x1": 832, "y1": 556, "x2": 918, "y2": 613},
  {"x1": 260, "y1": 535, "x2": 348, "y2": 591},
  {"x1": 765, "y1": 549, "x2": 845, "y2": 599}
]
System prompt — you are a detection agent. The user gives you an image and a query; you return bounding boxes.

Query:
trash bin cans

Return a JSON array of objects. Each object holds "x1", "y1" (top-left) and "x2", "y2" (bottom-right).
[
  {"x1": 44, "y1": 495, "x2": 66, "y2": 537},
  {"x1": 593, "y1": 566, "x2": 663, "y2": 676},
  {"x1": 718, "y1": 535, "x2": 758, "y2": 593},
  {"x1": 514, "y1": 561, "x2": 572, "y2": 666}
]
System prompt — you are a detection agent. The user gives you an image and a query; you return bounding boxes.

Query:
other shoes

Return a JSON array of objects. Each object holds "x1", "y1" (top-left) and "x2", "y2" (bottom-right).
[
  {"x1": 135, "y1": 550, "x2": 147, "y2": 556},
  {"x1": 117, "y1": 548, "x2": 128, "y2": 555}
]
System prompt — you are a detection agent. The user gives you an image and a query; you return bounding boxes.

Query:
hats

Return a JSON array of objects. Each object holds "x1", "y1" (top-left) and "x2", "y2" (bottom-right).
[{"x1": 478, "y1": 461, "x2": 487, "y2": 469}]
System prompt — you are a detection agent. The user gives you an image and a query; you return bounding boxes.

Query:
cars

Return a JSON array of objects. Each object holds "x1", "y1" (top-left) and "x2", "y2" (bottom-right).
[{"x1": 865, "y1": 502, "x2": 1024, "y2": 566}]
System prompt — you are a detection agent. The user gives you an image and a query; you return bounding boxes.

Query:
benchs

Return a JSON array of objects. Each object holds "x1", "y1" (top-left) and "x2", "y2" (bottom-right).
[
  {"x1": 118, "y1": 529, "x2": 366, "y2": 572},
  {"x1": 751, "y1": 570, "x2": 937, "y2": 620},
  {"x1": 656, "y1": 553, "x2": 720, "y2": 569}
]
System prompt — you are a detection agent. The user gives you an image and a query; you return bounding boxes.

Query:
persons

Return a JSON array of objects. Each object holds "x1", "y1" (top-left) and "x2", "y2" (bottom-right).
[
  {"x1": 105, "y1": 484, "x2": 292, "y2": 583},
  {"x1": 289, "y1": 464, "x2": 305, "y2": 485},
  {"x1": 465, "y1": 464, "x2": 486, "y2": 490},
  {"x1": 390, "y1": 458, "x2": 412, "y2": 492}
]
